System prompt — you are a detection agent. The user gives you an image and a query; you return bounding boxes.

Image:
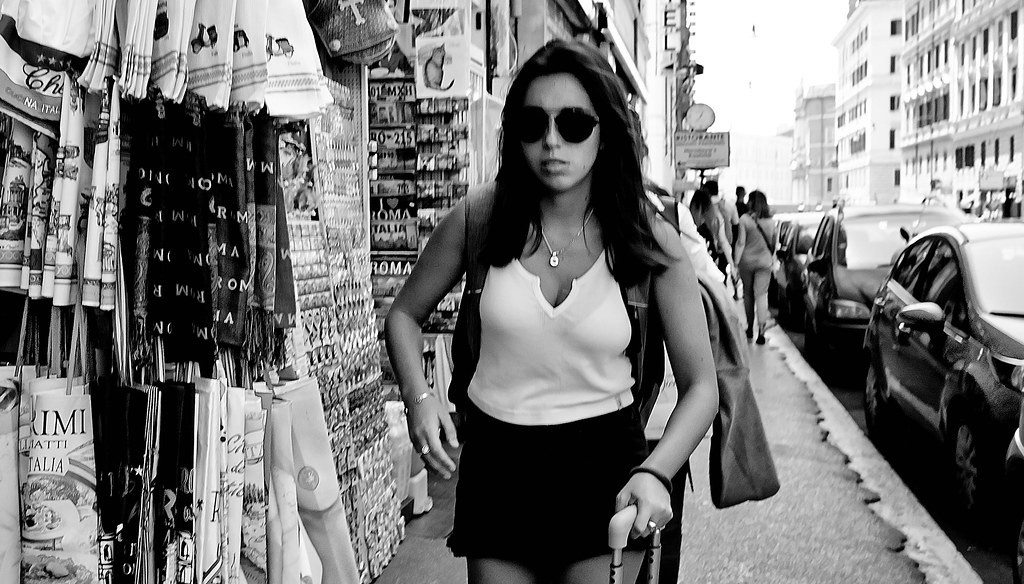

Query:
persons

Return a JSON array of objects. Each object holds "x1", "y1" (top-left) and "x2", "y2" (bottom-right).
[{"x1": 382, "y1": 37, "x2": 775, "y2": 584}]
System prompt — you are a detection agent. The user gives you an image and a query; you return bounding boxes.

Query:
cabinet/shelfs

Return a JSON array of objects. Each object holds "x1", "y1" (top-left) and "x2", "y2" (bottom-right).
[{"x1": 366, "y1": 77, "x2": 473, "y2": 259}]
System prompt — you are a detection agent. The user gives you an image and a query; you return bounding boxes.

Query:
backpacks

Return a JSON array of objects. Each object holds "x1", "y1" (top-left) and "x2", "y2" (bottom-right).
[{"x1": 697, "y1": 223, "x2": 718, "y2": 262}]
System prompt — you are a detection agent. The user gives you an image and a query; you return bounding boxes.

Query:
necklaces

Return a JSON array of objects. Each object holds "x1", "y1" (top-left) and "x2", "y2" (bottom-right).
[{"x1": 534, "y1": 207, "x2": 594, "y2": 267}]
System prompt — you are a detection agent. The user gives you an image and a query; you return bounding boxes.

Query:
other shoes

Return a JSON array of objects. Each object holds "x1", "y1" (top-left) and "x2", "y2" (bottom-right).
[
  {"x1": 756, "y1": 335, "x2": 766, "y2": 344},
  {"x1": 733, "y1": 296, "x2": 738, "y2": 301},
  {"x1": 745, "y1": 329, "x2": 754, "y2": 338}
]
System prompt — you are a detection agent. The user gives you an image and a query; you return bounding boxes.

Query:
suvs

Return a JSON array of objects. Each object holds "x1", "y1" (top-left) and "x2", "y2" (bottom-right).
[{"x1": 802, "y1": 197, "x2": 979, "y2": 408}]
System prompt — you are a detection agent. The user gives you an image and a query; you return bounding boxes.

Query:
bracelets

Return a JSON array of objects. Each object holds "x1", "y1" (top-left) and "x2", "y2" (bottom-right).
[
  {"x1": 629, "y1": 466, "x2": 673, "y2": 494},
  {"x1": 402, "y1": 389, "x2": 435, "y2": 415}
]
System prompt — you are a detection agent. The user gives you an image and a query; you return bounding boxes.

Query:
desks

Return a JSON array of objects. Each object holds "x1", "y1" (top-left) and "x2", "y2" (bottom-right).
[{"x1": 417, "y1": 333, "x2": 455, "y2": 418}]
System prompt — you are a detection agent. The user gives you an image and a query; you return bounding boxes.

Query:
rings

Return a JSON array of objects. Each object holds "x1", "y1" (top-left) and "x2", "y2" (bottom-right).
[
  {"x1": 421, "y1": 444, "x2": 431, "y2": 455},
  {"x1": 647, "y1": 519, "x2": 657, "y2": 534}
]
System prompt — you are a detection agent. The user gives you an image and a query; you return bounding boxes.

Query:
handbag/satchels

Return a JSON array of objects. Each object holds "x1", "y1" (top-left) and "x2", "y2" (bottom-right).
[{"x1": 0, "y1": 296, "x2": 310, "y2": 584}]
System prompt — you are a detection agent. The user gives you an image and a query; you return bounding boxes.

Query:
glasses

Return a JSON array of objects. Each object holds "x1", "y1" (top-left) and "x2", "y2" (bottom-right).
[{"x1": 516, "y1": 104, "x2": 597, "y2": 144}]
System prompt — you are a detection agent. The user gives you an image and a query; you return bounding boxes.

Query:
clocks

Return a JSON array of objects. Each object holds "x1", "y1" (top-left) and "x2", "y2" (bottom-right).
[{"x1": 686, "y1": 104, "x2": 715, "y2": 130}]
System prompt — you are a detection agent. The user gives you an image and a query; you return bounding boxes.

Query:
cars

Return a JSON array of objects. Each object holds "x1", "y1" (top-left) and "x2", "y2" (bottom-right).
[
  {"x1": 862, "y1": 220, "x2": 1024, "y2": 541},
  {"x1": 769, "y1": 211, "x2": 824, "y2": 332},
  {"x1": 1001, "y1": 393, "x2": 1024, "y2": 584}
]
